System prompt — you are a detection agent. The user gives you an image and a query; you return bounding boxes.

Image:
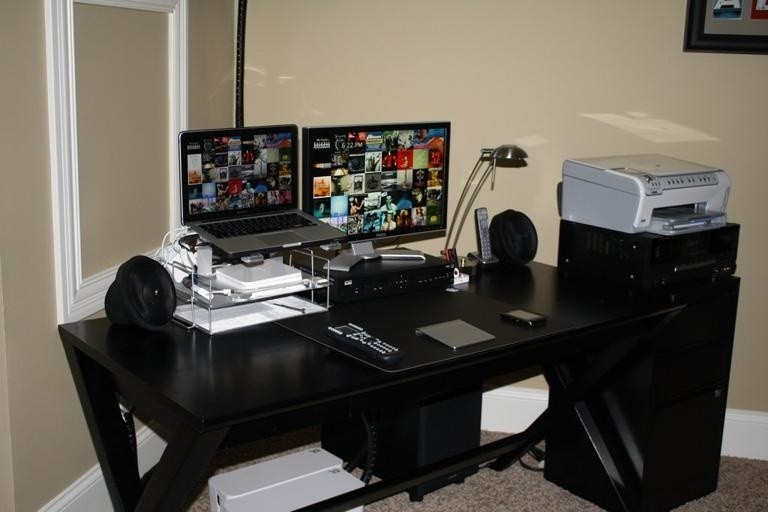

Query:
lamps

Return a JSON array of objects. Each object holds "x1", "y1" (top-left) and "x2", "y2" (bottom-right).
[{"x1": 441, "y1": 141, "x2": 531, "y2": 272}]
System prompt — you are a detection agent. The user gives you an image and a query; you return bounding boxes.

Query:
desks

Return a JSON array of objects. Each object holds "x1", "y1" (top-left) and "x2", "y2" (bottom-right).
[{"x1": 56, "y1": 249, "x2": 694, "y2": 512}]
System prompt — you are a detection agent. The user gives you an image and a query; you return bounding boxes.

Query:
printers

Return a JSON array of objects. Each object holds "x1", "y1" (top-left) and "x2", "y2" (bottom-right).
[{"x1": 562, "y1": 154, "x2": 730, "y2": 237}]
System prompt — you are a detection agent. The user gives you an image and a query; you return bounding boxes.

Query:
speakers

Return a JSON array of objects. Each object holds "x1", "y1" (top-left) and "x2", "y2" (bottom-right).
[
  {"x1": 543, "y1": 267, "x2": 740, "y2": 512},
  {"x1": 323, "y1": 393, "x2": 481, "y2": 501}
]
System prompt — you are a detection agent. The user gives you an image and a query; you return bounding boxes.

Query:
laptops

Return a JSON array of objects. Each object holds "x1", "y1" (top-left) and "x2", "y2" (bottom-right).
[{"x1": 179, "y1": 124, "x2": 349, "y2": 260}]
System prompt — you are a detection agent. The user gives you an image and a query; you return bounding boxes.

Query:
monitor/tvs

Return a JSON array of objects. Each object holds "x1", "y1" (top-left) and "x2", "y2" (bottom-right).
[{"x1": 302, "y1": 122, "x2": 450, "y2": 272}]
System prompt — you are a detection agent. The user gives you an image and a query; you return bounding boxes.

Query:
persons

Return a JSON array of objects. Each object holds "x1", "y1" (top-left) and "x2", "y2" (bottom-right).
[
  {"x1": 243, "y1": 150, "x2": 254, "y2": 162},
  {"x1": 411, "y1": 208, "x2": 424, "y2": 227},
  {"x1": 386, "y1": 136, "x2": 392, "y2": 151},
  {"x1": 406, "y1": 135, "x2": 413, "y2": 150},
  {"x1": 380, "y1": 196, "x2": 397, "y2": 211},
  {"x1": 217, "y1": 186, "x2": 228, "y2": 196},
  {"x1": 255, "y1": 194, "x2": 266, "y2": 206},
  {"x1": 319, "y1": 200, "x2": 326, "y2": 215},
  {"x1": 350, "y1": 202, "x2": 364, "y2": 214},
  {"x1": 382, "y1": 213, "x2": 396, "y2": 231},
  {"x1": 268, "y1": 191, "x2": 278, "y2": 204},
  {"x1": 413, "y1": 129, "x2": 428, "y2": 144}
]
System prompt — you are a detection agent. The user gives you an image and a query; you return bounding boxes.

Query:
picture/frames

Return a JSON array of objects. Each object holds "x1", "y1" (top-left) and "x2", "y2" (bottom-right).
[{"x1": 680, "y1": 0, "x2": 767, "y2": 57}]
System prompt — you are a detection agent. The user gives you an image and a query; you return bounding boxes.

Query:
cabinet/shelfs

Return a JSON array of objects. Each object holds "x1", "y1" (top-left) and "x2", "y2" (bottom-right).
[{"x1": 540, "y1": 217, "x2": 744, "y2": 511}]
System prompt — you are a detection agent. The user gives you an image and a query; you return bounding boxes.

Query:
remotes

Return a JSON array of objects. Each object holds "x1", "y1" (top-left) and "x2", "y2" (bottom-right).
[
  {"x1": 325, "y1": 321, "x2": 405, "y2": 366},
  {"x1": 475, "y1": 208, "x2": 492, "y2": 261}
]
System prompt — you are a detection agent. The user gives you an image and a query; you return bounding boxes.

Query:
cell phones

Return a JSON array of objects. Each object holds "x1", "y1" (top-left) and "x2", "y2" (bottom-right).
[{"x1": 499, "y1": 306, "x2": 547, "y2": 326}]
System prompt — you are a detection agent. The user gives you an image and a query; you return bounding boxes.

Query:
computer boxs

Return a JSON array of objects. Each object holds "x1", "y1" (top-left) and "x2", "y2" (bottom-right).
[{"x1": 286, "y1": 248, "x2": 453, "y2": 304}]
